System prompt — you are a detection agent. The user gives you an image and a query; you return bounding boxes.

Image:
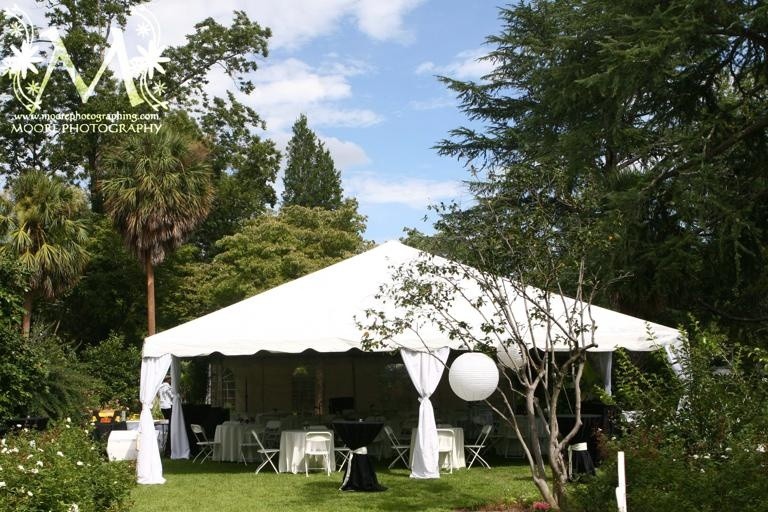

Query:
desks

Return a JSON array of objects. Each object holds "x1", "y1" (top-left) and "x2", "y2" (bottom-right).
[{"x1": 87, "y1": 418, "x2": 172, "y2": 463}]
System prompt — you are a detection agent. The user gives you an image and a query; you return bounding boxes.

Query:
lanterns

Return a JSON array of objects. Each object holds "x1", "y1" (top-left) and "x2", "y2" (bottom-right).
[{"x1": 448, "y1": 352, "x2": 499, "y2": 403}]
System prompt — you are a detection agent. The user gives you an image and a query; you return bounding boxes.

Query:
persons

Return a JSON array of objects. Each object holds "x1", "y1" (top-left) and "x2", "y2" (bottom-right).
[{"x1": 156, "y1": 374, "x2": 174, "y2": 420}]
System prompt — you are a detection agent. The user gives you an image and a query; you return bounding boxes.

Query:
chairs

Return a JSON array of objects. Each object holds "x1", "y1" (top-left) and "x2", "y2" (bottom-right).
[{"x1": 189, "y1": 412, "x2": 541, "y2": 479}]
